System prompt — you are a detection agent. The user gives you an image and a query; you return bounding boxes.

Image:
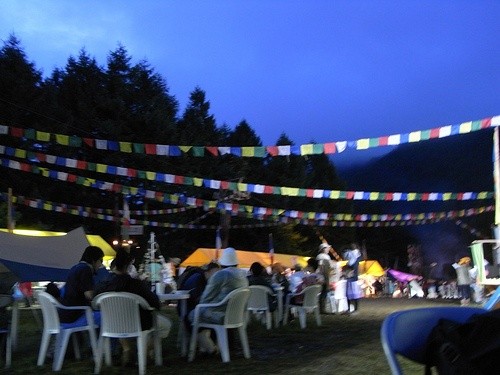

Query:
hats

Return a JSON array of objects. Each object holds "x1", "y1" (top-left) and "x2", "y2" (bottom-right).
[
  {"x1": 220, "y1": 248, "x2": 239, "y2": 266},
  {"x1": 317, "y1": 243, "x2": 331, "y2": 252}
]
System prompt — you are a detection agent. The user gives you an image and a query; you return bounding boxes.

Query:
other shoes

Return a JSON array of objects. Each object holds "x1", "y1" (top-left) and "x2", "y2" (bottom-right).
[
  {"x1": 146, "y1": 356, "x2": 155, "y2": 364},
  {"x1": 120, "y1": 351, "x2": 134, "y2": 367}
]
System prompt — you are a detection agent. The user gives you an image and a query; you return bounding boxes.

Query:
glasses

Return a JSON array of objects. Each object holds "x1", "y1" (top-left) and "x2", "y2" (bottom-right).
[{"x1": 98, "y1": 259, "x2": 102, "y2": 263}]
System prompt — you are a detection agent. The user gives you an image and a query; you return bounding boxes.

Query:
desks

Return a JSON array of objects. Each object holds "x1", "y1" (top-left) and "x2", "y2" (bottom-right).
[{"x1": 154, "y1": 292, "x2": 190, "y2": 366}]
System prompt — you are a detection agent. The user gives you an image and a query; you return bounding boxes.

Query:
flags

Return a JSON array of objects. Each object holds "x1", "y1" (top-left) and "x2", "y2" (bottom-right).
[{"x1": 0, "y1": 116, "x2": 500, "y2": 240}]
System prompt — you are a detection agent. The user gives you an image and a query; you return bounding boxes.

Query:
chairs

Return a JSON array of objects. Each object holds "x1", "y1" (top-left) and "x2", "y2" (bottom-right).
[
  {"x1": 379, "y1": 307, "x2": 487, "y2": 375},
  {"x1": 0, "y1": 280, "x2": 347, "y2": 375}
]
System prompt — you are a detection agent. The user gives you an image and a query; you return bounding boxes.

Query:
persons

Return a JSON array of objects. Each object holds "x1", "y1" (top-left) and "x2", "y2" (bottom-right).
[
  {"x1": 19, "y1": 245, "x2": 366, "y2": 365},
  {"x1": 376, "y1": 258, "x2": 499, "y2": 306}
]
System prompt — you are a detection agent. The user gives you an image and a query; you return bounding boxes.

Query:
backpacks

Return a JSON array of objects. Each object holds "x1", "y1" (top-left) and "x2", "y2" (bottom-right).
[{"x1": 176, "y1": 267, "x2": 200, "y2": 290}]
